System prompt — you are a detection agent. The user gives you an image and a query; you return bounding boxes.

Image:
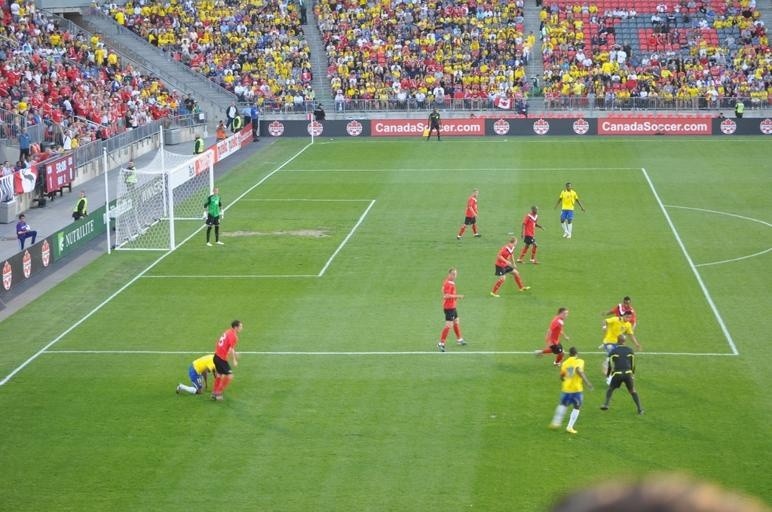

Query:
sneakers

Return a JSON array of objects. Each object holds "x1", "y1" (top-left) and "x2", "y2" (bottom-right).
[
  {"x1": 216, "y1": 241, "x2": 224, "y2": 245},
  {"x1": 176, "y1": 383, "x2": 182, "y2": 394},
  {"x1": 211, "y1": 393, "x2": 223, "y2": 401},
  {"x1": 207, "y1": 242, "x2": 212, "y2": 247}
]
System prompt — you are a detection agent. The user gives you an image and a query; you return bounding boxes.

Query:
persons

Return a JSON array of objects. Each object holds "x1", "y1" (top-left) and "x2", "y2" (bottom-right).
[
  {"x1": 538, "y1": 1, "x2": 771, "y2": 118},
  {"x1": 210, "y1": 320, "x2": 244, "y2": 402},
  {"x1": 16, "y1": 213, "x2": 38, "y2": 250},
  {"x1": 251, "y1": 102, "x2": 263, "y2": 142},
  {"x1": 599, "y1": 310, "x2": 641, "y2": 356},
  {"x1": 552, "y1": 181, "x2": 587, "y2": 240},
  {"x1": 437, "y1": 268, "x2": 468, "y2": 353},
  {"x1": 426, "y1": 107, "x2": 444, "y2": 143},
  {"x1": 71, "y1": 190, "x2": 89, "y2": 221},
  {"x1": 175, "y1": 355, "x2": 218, "y2": 395},
  {"x1": 1, "y1": 0, "x2": 205, "y2": 209},
  {"x1": 533, "y1": 306, "x2": 572, "y2": 371},
  {"x1": 490, "y1": 234, "x2": 531, "y2": 299},
  {"x1": 548, "y1": 347, "x2": 595, "y2": 435},
  {"x1": 202, "y1": 0, "x2": 541, "y2": 133},
  {"x1": 201, "y1": 187, "x2": 225, "y2": 248},
  {"x1": 215, "y1": 119, "x2": 227, "y2": 142},
  {"x1": 516, "y1": 206, "x2": 547, "y2": 265},
  {"x1": 455, "y1": 187, "x2": 484, "y2": 241},
  {"x1": 603, "y1": 296, "x2": 637, "y2": 345},
  {"x1": 598, "y1": 334, "x2": 645, "y2": 416}
]
